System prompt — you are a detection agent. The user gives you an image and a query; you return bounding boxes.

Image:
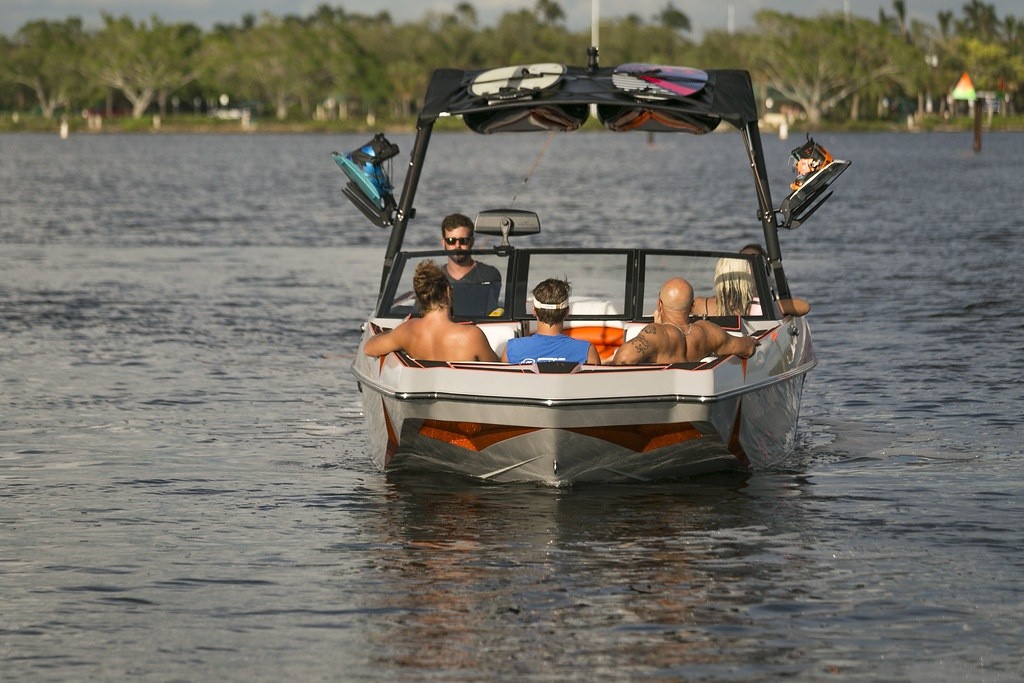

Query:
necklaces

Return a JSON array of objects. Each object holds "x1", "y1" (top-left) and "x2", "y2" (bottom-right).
[{"x1": 663, "y1": 323, "x2": 691, "y2": 335}]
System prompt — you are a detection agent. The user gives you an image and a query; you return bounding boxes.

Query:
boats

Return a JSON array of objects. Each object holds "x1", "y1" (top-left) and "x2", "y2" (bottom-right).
[{"x1": 331, "y1": 47, "x2": 852, "y2": 488}]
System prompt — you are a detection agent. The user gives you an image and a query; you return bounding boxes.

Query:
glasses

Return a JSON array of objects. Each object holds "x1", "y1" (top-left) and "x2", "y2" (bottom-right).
[{"x1": 444, "y1": 237, "x2": 471, "y2": 245}]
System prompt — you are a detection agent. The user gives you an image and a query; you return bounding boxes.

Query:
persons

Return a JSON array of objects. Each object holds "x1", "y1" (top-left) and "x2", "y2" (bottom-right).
[
  {"x1": 500, "y1": 279, "x2": 601, "y2": 365},
  {"x1": 612, "y1": 278, "x2": 761, "y2": 366},
  {"x1": 363, "y1": 259, "x2": 500, "y2": 362},
  {"x1": 410, "y1": 213, "x2": 502, "y2": 319},
  {"x1": 690, "y1": 245, "x2": 810, "y2": 317}
]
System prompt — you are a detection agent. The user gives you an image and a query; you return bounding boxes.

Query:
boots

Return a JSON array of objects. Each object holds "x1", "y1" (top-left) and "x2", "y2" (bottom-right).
[
  {"x1": 330, "y1": 132, "x2": 401, "y2": 228},
  {"x1": 790, "y1": 132, "x2": 834, "y2": 190}
]
